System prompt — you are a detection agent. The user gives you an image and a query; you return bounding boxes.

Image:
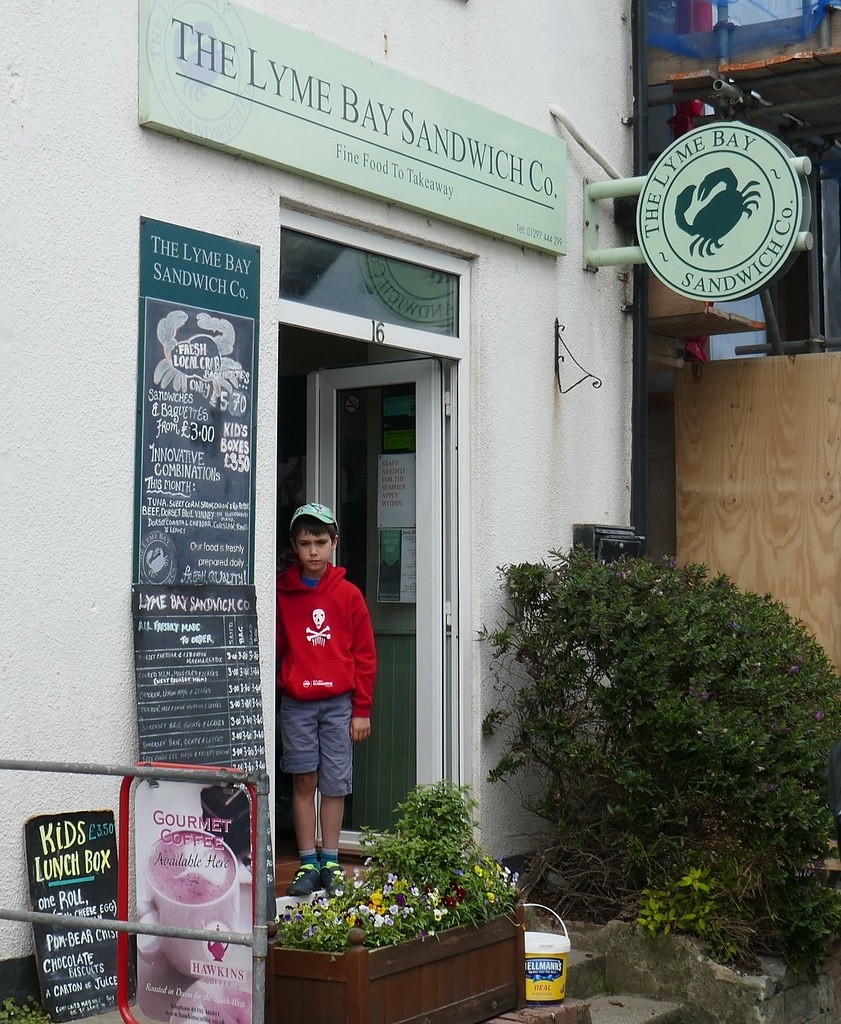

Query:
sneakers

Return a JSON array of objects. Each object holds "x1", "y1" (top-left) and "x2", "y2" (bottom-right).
[
  {"x1": 286, "y1": 865, "x2": 321, "y2": 896},
  {"x1": 320, "y1": 861, "x2": 345, "y2": 896}
]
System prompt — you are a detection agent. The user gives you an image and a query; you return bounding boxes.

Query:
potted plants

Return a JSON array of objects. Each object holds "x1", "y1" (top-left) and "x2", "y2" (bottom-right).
[{"x1": 275, "y1": 778, "x2": 526, "y2": 1024}]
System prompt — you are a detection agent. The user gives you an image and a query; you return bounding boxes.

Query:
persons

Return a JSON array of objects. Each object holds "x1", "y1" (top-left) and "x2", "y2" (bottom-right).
[{"x1": 276, "y1": 503, "x2": 377, "y2": 896}]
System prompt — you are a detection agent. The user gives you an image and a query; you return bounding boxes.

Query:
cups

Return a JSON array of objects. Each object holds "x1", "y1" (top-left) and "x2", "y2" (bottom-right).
[{"x1": 133, "y1": 828, "x2": 239, "y2": 979}]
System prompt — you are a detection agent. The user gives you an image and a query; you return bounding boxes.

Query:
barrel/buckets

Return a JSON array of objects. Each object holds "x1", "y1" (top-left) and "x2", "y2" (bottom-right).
[{"x1": 518, "y1": 902, "x2": 572, "y2": 1008}]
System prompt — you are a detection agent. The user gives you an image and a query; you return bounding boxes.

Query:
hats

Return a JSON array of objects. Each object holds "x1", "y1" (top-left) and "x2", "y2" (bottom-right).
[{"x1": 290, "y1": 503, "x2": 339, "y2": 534}]
